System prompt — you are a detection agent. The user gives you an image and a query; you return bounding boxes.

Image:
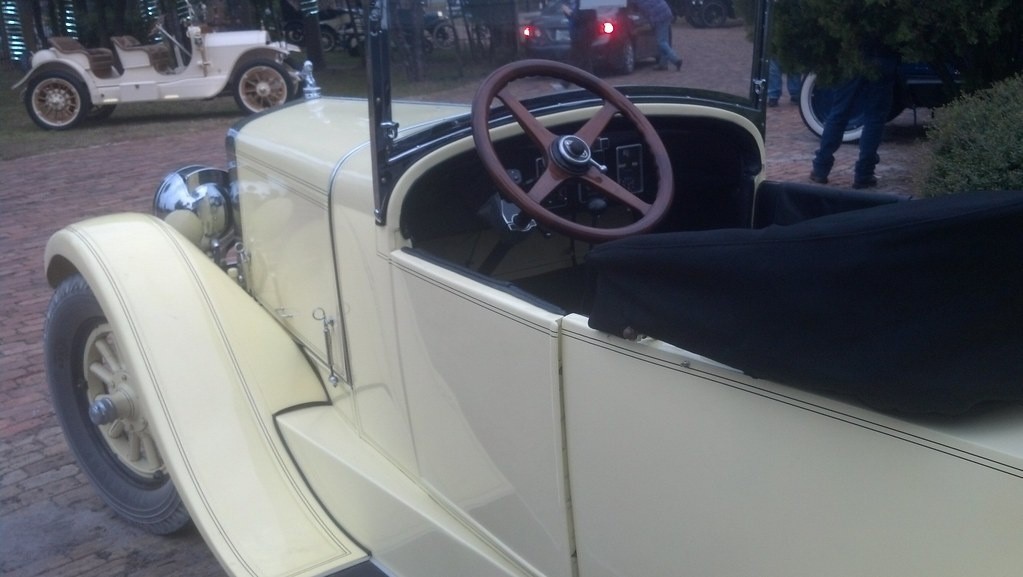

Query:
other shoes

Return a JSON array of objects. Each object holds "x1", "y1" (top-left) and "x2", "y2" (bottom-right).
[
  {"x1": 768, "y1": 99, "x2": 778, "y2": 107},
  {"x1": 550, "y1": 83, "x2": 564, "y2": 92},
  {"x1": 653, "y1": 64, "x2": 668, "y2": 70},
  {"x1": 810, "y1": 168, "x2": 827, "y2": 183},
  {"x1": 791, "y1": 95, "x2": 798, "y2": 104},
  {"x1": 852, "y1": 175, "x2": 877, "y2": 189},
  {"x1": 676, "y1": 59, "x2": 683, "y2": 70}
]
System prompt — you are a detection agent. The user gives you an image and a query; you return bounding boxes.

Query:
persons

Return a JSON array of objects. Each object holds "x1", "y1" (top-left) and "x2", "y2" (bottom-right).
[
  {"x1": 767, "y1": 56, "x2": 801, "y2": 107},
  {"x1": 631, "y1": 1, "x2": 683, "y2": 72},
  {"x1": 550, "y1": 0, "x2": 596, "y2": 91},
  {"x1": 809, "y1": 42, "x2": 896, "y2": 188}
]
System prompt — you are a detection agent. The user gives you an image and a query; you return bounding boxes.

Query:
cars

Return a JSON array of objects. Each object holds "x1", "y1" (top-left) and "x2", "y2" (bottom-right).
[
  {"x1": 676, "y1": 1, "x2": 742, "y2": 28},
  {"x1": 516, "y1": 0, "x2": 676, "y2": 78},
  {"x1": 286, "y1": 6, "x2": 463, "y2": 56},
  {"x1": 45, "y1": 61, "x2": 1022, "y2": 574},
  {"x1": 11, "y1": 7, "x2": 315, "y2": 130},
  {"x1": 796, "y1": 53, "x2": 978, "y2": 145}
]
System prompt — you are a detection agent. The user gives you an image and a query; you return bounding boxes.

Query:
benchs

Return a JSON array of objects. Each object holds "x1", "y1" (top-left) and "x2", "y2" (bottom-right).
[
  {"x1": 47, "y1": 36, "x2": 115, "y2": 71},
  {"x1": 110, "y1": 34, "x2": 170, "y2": 69},
  {"x1": 582, "y1": 181, "x2": 1023, "y2": 419}
]
